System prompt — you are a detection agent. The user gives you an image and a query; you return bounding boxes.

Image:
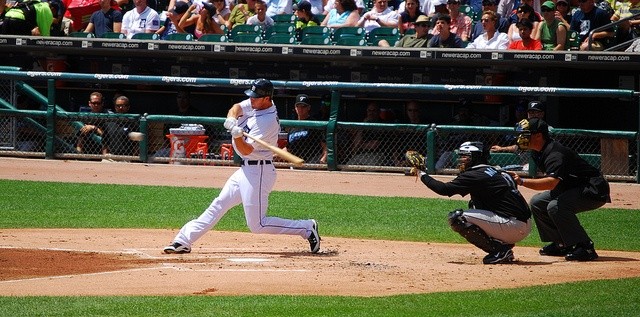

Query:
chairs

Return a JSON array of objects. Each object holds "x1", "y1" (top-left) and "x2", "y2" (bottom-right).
[
  {"x1": 233, "y1": 35, "x2": 262, "y2": 43},
  {"x1": 300, "y1": 26, "x2": 331, "y2": 36},
  {"x1": 301, "y1": 36, "x2": 332, "y2": 45},
  {"x1": 269, "y1": 23, "x2": 296, "y2": 33},
  {"x1": 460, "y1": 6, "x2": 474, "y2": 28},
  {"x1": 165, "y1": 33, "x2": 192, "y2": 41},
  {"x1": 565, "y1": 30, "x2": 580, "y2": 51},
  {"x1": 198, "y1": 35, "x2": 228, "y2": 42},
  {"x1": 272, "y1": 14, "x2": 293, "y2": 22},
  {"x1": 131, "y1": 33, "x2": 160, "y2": 40},
  {"x1": 69, "y1": 32, "x2": 95, "y2": 38},
  {"x1": 232, "y1": 26, "x2": 262, "y2": 35},
  {"x1": 335, "y1": 36, "x2": 367, "y2": 46},
  {"x1": 334, "y1": 27, "x2": 366, "y2": 35},
  {"x1": 100, "y1": 32, "x2": 127, "y2": 38},
  {"x1": 369, "y1": 27, "x2": 402, "y2": 37},
  {"x1": 294, "y1": 15, "x2": 326, "y2": 22},
  {"x1": 268, "y1": 34, "x2": 296, "y2": 44},
  {"x1": 368, "y1": 37, "x2": 402, "y2": 47}
]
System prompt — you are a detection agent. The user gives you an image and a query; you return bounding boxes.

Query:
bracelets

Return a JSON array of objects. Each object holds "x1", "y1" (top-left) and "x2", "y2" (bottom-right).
[
  {"x1": 216, "y1": 14, "x2": 222, "y2": 16},
  {"x1": 519, "y1": 178, "x2": 524, "y2": 185},
  {"x1": 375, "y1": 17, "x2": 380, "y2": 22}
]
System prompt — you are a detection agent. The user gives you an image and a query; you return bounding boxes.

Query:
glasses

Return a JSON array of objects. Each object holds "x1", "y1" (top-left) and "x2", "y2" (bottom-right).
[
  {"x1": 115, "y1": 104, "x2": 128, "y2": 107},
  {"x1": 448, "y1": 1, "x2": 458, "y2": 4},
  {"x1": 517, "y1": 8, "x2": 528, "y2": 13},
  {"x1": 407, "y1": 108, "x2": 419, "y2": 112},
  {"x1": 90, "y1": 101, "x2": 103, "y2": 105},
  {"x1": 420, "y1": 23, "x2": 431, "y2": 27},
  {"x1": 480, "y1": 18, "x2": 491, "y2": 22},
  {"x1": 542, "y1": 8, "x2": 552, "y2": 12},
  {"x1": 558, "y1": 2, "x2": 567, "y2": 6}
]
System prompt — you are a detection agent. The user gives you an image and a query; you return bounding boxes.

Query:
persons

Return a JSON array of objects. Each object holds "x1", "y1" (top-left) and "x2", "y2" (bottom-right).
[
  {"x1": 431, "y1": 0, "x2": 472, "y2": 41},
  {"x1": 266, "y1": 0, "x2": 293, "y2": 16},
  {"x1": 247, "y1": 0, "x2": 274, "y2": 25},
  {"x1": 496, "y1": 0, "x2": 542, "y2": 17},
  {"x1": 502, "y1": 101, "x2": 528, "y2": 126},
  {"x1": 320, "y1": 0, "x2": 360, "y2": 27},
  {"x1": 161, "y1": 76, "x2": 323, "y2": 254},
  {"x1": 0, "y1": 0, "x2": 67, "y2": 38},
  {"x1": 0, "y1": 90, "x2": 47, "y2": 152},
  {"x1": 605, "y1": 0, "x2": 624, "y2": 10},
  {"x1": 209, "y1": 0, "x2": 230, "y2": 26},
  {"x1": 533, "y1": 0, "x2": 568, "y2": 52},
  {"x1": 413, "y1": 140, "x2": 532, "y2": 265},
  {"x1": 396, "y1": 0, "x2": 429, "y2": 35},
  {"x1": 556, "y1": 0, "x2": 573, "y2": 25},
  {"x1": 427, "y1": 14, "x2": 467, "y2": 48},
  {"x1": 472, "y1": 0, "x2": 510, "y2": 38},
  {"x1": 225, "y1": 0, "x2": 261, "y2": 30},
  {"x1": 440, "y1": 103, "x2": 499, "y2": 165},
  {"x1": 566, "y1": 0, "x2": 615, "y2": 52},
  {"x1": 354, "y1": 0, "x2": 400, "y2": 27},
  {"x1": 120, "y1": 0, "x2": 161, "y2": 35},
  {"x1": 504, "y1": 118, "x2": 611, "y2": 262},
  {"x1": 155, "y1": 0, "x2": 190, "y2": 41},
  {"x1": 490, "y1": 99, "x2": 549, "y2": 153},
  {"x1": 271, "y1": 92, "x2": 328, "y2": 165},
  {"x1": 609, "y1": 0, "x2": 640, "y2": 34},
  {"x1": 322, "y1": 0, "x2": 366, "y2": 15},
  {"x1": 506, "y1": 3, "x2": 539, "y2": 42},
  {"x1": 291, "y1": 0, "x2": 321, "y2": 29},
  {"x1": 509, "y1": 0, "x2": 543, "y2": 22},
  {"x1": 69, "y1": 90, "x2": 111, "y2": 164},
  {"x1": 350, "y1": 98, "x2": 385, "y2": 146},
  {"x1": 83, "y1": 0, "x2": 122, "y2": 33},
  {"x1": 506, "y1": 18, "x2": 543, "y2": 51},
  {"x1": 178, "y1": 2, "x2": 224, "y2": 38},
  {"x1": 103, "y1": 94, "x2": 146, "y2": 158},
  {"x1": 167, "y1": 0, "x2": 203, "y2": 13},
  {"x1": 395, "y1": 101, "x2": 432, "y2": 167},
  {"x1": 464, "y1": 9, "x2": 511, "y2": 50},
  {"x1": 377, "y1": 14, "x2": 435, "y2": 48}
]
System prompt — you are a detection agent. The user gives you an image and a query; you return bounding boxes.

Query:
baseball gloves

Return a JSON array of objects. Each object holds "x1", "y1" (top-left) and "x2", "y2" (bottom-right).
[{"x1": 405, "y1": 150, "x2": 428, "y2": 176}]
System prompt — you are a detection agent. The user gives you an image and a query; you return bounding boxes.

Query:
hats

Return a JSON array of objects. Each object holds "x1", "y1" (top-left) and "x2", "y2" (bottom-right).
[
  {"x1": 481, "y1": 0, "x2": 497, "y2": 4},
  {"x1": 50, "y1": 1, "x2": 60, "y2": 18},
  {"x1": 244, "y1": 77, "x2": 274, "y2": 98},
  {"x1": 541, "y1": 0, "x2": 556, "y2": 9},
  {"x1": 295, "y1": 94, "x2": 312, "y2": 105},
  {"x1": 292, "y1": 0, "x2": 312, "y2": 11},
  {"x1": 202, "y1": 2, "x2": 217, "y2": 17},
  {"x1": 411, "y1": 13, "x2": 433, "y2": 24},
  {"x1": 169, "y1": 0, "x2": 189, "y2": 14},
  {"x1": 528, "y1": 100, "x2": 544, "y2": 111}
]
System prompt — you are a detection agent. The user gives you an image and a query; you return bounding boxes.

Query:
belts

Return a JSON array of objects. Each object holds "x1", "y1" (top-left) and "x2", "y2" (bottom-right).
[{"x1": 240, "y1": 159, "x2": 273, "y2": 164}]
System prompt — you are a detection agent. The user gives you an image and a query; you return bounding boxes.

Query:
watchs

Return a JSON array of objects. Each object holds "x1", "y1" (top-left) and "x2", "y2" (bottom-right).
[{"x1": 243, "y1": 11, "x2": 250, "y2": 16}]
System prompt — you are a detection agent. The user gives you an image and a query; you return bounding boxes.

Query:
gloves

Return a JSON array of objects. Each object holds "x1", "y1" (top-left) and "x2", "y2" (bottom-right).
[
  {"x1": 223, "y1": 116, "x2": 239, "y2": 130},
  {"x1": 230, "y1": 126, "x2": 245, "y2": 138}
]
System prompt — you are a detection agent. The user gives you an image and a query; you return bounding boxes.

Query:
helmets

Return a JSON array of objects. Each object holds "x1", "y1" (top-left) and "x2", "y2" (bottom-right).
[
  {"x1": 514, "y1": 117, "x2": 549, "y2": 152},
  {"x1": 454, "y1": 140, "x2": 492, "y2": 172}
]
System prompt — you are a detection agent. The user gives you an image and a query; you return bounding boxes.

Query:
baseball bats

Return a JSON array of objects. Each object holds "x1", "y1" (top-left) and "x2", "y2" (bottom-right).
[{"x1": 242, "y1": 130, "x2": 305, "y2": 167}]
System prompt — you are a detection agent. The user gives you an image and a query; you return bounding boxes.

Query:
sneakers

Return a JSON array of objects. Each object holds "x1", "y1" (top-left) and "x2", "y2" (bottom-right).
[
  {"x1": 540, "y1": 241, "x2": 575, "y2": 256},
  {"x1": 306, "y1": 218, "x2": 321, "y2": 253},
  {"x1": 565, "y1": 241, "x2": 599, "y2": 260},
  {"x1": 482, "y1": 246, "x2": 514, "y2": 264},
  {"x1": 163, "y1": 241, "x2": 191, "y2": 253}
]
